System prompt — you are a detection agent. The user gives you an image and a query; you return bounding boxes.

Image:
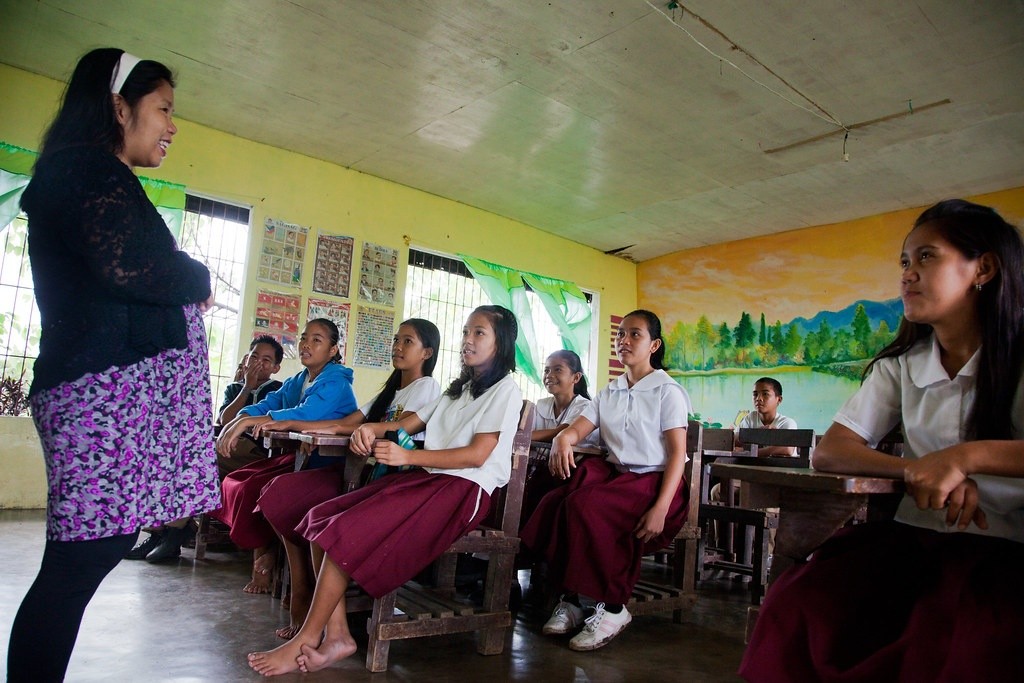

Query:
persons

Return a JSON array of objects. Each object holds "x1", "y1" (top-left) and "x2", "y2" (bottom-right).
[
  {"x1": 123, "y1": 305, "x2": 688, "y2": 675},
  {"x1": 710, "y1": 376, "x2": 797, "y2": 583},
  {"x1": 6, "y1": 47, "x2": 215, "y2": 683},
  {"x1": 740, "y1": 197, "x2": 1023, "y2": 681}
]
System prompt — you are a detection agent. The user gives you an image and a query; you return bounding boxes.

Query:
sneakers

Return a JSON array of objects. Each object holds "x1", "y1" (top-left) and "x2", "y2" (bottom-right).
[
  {"x1": 543, "y1": 594, "x2": 584, "y2": 633},
  {"x1": 568, "y1": 601, "x2": 633, "y2": 650}
]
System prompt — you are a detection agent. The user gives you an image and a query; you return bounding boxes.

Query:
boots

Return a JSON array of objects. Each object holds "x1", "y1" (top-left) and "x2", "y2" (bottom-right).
[
  {"x1": 122, "y1": 530, "x2": 164, "y2": 560},
  {"x1": 145, "y1": 525, "x2": 183, "y2": 562}
]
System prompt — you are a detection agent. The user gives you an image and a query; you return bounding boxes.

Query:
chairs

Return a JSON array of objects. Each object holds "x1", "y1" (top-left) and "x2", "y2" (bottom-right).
[
  {"x1": 700, "y1": 424, "x2": 735, "y2": 469},
  {"x1": 184, "y1": 399, "x2": 535, "y2": 673},
  {"x1": 708, "y1": 427, "x2": 816, "y2": 605},
  {"x1": 623, "y1": 417, "x2": 705, "y2": 624}
]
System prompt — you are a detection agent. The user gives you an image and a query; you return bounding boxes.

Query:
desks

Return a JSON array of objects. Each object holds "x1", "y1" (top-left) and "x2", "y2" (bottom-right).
[
  {"x1": 710, "y1": 461, "x2": 902, "y2": 594},
  {"x1": 529, "y1": 440, "x2": 604, "y2": 479},
  {"x1": 245, "y1": 426, "x2": 294, "y2": 458},
  {"x1": 287, "y1": 428, "x2": 389, "y2": 460}
]
